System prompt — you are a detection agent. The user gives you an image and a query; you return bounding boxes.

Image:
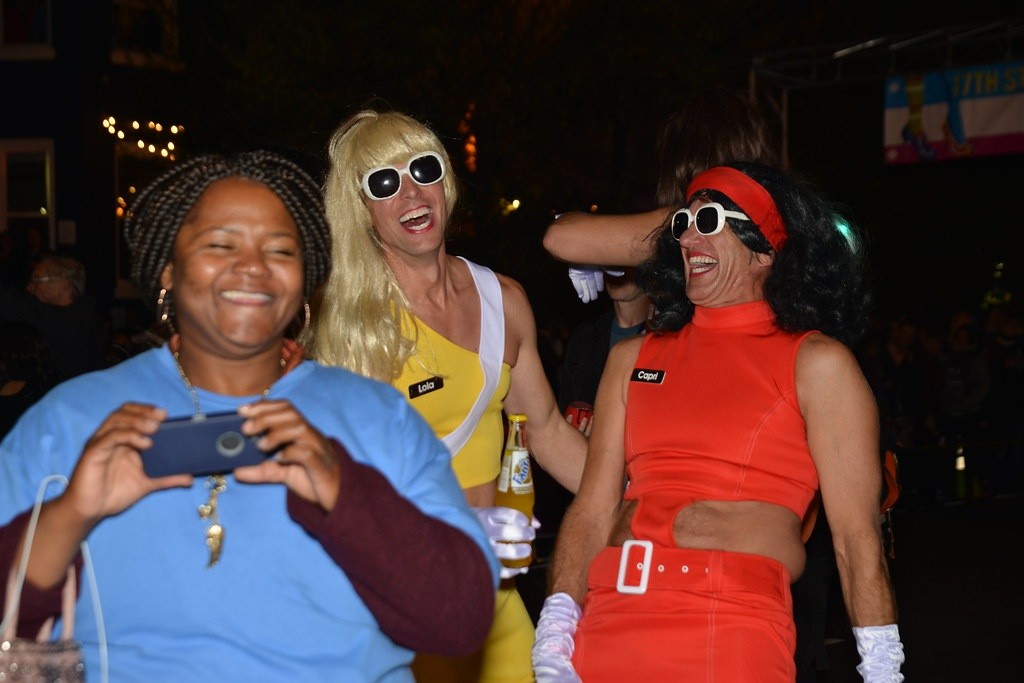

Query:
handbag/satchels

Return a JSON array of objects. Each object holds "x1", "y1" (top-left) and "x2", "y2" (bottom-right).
[{"x1": 0, "y1": 506, "x2": 84, "y2": 683}]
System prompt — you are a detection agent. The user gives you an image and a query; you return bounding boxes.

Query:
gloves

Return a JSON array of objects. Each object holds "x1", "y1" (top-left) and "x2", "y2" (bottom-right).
[
  {"x1": 555, "y1": 215, "x2": 625, "y2": 304},
  {"x1": 472, "y1": 505, "x2": 541, "y2": 579}
]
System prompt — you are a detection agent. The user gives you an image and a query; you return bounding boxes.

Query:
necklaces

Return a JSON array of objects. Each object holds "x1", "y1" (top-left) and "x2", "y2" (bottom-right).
[{"x1": 172, "y1": 325, "x2": 301, "y2": 566}]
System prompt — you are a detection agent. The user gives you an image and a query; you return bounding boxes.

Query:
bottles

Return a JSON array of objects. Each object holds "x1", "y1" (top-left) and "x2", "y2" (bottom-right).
[
  {"x1": 492, "y1": 411, "x2": 534, "y2": 567},
  {"x1": 953, "y1": 434, "x2": 967, "y2": 498},
  {"x1": 884, "y1": 507, "x2": 899, "y2": 561}
]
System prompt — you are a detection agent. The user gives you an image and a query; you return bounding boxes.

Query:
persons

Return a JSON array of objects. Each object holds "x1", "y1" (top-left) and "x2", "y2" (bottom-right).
[
  {"x1": 0, "y1": 237, "x2": 173, "y2": 441},
  {"x1": 0, "y1": 150, "x2": 499, "y2": 683},
  {"x1": 502, "y1": 86, "x2": 1024, "y2": 683},
  {"x1": 528, "y1": 168, "x2": 910, "y2": 683},
  {"x1": 301, "y1": 110, "x2": 589, "y2": 683}
]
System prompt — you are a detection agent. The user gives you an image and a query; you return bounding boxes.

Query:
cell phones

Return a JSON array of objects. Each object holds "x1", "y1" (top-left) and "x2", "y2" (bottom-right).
[{"x1": 140, "y1": 409, "x2": 269, "y2": 479}]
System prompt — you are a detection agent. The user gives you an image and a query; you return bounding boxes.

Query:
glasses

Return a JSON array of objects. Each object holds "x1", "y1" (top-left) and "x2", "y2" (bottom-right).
[
  {"x1": 360, "y1": 151, "x2": 446, "y2": 200},
  {"x1": 670, "y1": 202, "x2": 750, "y2": 241}
]
